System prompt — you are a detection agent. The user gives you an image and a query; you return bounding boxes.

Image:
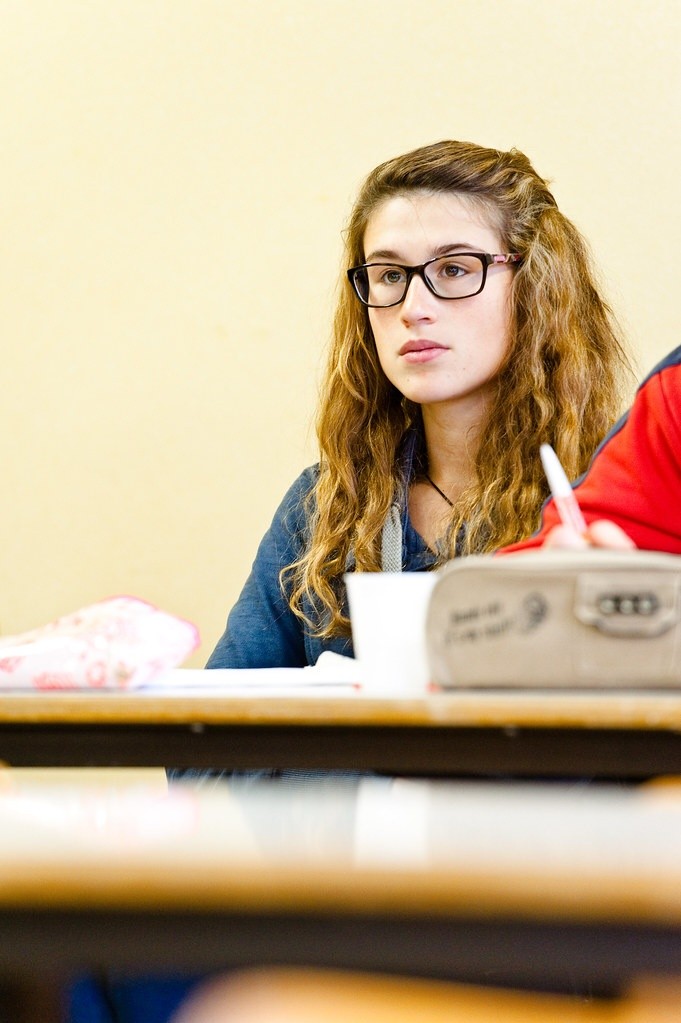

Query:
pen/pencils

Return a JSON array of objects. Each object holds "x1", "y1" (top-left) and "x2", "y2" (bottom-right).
[{"x1": 540, "y1": 441, "x2": 587, "y2": 538}]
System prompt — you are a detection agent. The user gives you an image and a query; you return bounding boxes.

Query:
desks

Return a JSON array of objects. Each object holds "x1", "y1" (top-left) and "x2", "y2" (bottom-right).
[{"x1": 0, "y1": 680, "x2": 681, "y2": 1023}]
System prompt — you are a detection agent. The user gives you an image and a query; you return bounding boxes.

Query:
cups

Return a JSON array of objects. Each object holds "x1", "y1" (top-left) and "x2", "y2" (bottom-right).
[{"x1": 342, "y1": 571, "x2": 437, "y2": 692}]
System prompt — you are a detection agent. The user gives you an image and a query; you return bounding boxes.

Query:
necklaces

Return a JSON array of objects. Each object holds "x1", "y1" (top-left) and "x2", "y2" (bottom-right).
[{"x1": 423, "y1": 472, "x2": 466, "y2": 523}]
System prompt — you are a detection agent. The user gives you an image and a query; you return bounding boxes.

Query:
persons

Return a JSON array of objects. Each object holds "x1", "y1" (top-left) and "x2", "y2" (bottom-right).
[
  {"x1": 494, "y1": 346, "x2": 680, "y2": 556},
  {"x1": 164, "y1": 141, "x2": 681, "y2": 791}
]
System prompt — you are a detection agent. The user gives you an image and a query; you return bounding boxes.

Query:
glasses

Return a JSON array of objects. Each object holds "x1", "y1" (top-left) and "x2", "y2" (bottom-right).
[{"x1": 346, "y1": 251, "x2": 525, "y2": 309}]
